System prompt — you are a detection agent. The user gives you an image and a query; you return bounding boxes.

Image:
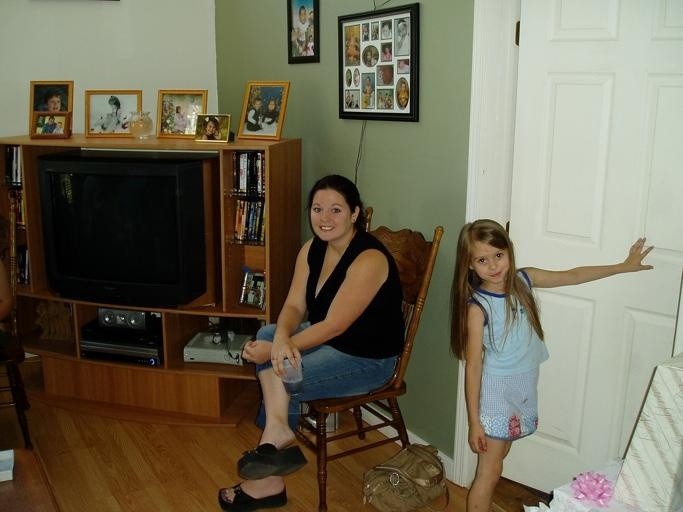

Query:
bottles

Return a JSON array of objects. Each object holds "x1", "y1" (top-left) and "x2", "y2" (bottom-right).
[{"x1": 130, "y1": 112, "x2": 153, "y2": 140}]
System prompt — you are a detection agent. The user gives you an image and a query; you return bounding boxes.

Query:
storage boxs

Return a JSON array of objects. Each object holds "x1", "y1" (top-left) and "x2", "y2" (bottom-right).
[
  {"x1": 549, "y1": 459, "x2": 641, "y2": 512},
  {"x1": 610, "y1": 351, "x2": 683, "y2": 511}
]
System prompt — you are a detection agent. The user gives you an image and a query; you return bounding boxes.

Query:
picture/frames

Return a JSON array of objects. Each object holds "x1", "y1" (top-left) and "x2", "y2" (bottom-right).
[
  {"x1": 192, "y1": 113, "x2": 231, "y2": 143},
  {"x1": 286, "y1": 0, "x2": 321, "y2": 65},
  {"x1": 29, "y1": 111, "x2": 72, "y2": 140},
  {"x1": 154, "y1": 88, "x2": 209, "y2": 140},
  {"x1": 83, "y1": 89, "x2": 144, "y2": 140},
  {"x1": 26, "y1": 77, "x2": 75, "y2": 133},
  {"x1": 338, "y1": 2, "x2": 422, "y2": 122},
  {"x1": 235, "y1": 78, "x2": 292, "y2": 142}
]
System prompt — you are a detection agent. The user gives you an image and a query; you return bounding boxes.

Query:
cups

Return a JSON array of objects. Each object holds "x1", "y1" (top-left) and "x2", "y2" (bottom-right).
[{"x1": 283, "y1": 354, "x2": 304, "y2": 384}]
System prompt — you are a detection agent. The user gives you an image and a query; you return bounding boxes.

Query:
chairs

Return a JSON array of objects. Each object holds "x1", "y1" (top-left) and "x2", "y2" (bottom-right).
[{"x1": 290, "y1": 206, "x2": 445, "y2": 512}]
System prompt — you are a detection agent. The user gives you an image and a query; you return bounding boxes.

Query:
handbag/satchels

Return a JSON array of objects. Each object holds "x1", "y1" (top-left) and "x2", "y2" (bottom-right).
[{"x1": 360, "y1": 444, "x2": 450, "y2": 512}]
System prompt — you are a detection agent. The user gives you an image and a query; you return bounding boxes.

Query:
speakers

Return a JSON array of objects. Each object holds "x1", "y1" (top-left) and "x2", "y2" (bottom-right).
[{"x1": 96, "y1": 307, "x2": 148, "y2": 334}]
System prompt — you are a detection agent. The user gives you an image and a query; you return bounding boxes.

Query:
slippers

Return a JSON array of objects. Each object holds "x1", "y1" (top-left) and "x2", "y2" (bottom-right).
[
  {"x1": 218, "y1": 483, "x2": 290, "y2": 511},
  {"x1": 237, "y1": 442, "x2": 309, "y2": 482}
]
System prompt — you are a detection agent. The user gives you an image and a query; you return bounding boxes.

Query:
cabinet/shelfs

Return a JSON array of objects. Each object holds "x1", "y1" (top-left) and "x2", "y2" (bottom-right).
[{"x1": 0, "y1": 134, "x2": 303, "y2": 433}]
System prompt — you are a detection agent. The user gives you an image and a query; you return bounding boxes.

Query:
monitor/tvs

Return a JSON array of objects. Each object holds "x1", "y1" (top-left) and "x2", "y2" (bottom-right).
[{"x1": 36, "y1": 152, "x2": 207, "y2": 310}]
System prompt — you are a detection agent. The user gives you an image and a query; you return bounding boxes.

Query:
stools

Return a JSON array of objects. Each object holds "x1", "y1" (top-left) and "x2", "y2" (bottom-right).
[{"x1": 1, "y1": 331, "x2": 35, "y2": 453}]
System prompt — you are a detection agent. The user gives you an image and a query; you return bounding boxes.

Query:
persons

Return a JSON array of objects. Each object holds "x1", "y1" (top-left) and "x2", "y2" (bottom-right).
[
  {"x1": 42, "y1": 116, "x2": 57, "y2": 133},
  {"x1": 345, "y1": 20, "x2": 408, "y2": 109},
  {"x1": 202, "y1": 117, "x2": 222, "y2": 140},
  {"x1": 292, "y1": 5, "x2": 314, "y2": 57},
  {"x1": 247, "y1": 98, "x2": 263, "y2": 131},
  {"x1": 261, "y1": 98, "x2": 278, "y2": 128},
  {"x1": 45, "y1": 92, "x2": 62, "y2": 111},
  {"x1": 103, "y1": 96, "x2": 123, "y2": 133},
  {"x1": 52, "y1": 122, "x2": 64, "y2": 134},
  {"x1": 451, "y1": 219, "x2": 653, "y2": 512},
  {"x1": 0, "y1": 259, "x2": 15, "y2": 352},
  {"x1": 172, "y1": 105, "x2": 187, "y2": 132},
  {"x1": 218, "y1": 176, "x2": 405, "y2": 511}
]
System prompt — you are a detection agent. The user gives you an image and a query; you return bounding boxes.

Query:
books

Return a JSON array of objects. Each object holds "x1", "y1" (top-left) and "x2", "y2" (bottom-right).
[
  {"x1": 6, "y1": 146, "x2": 29, "y2": 285},
  {"x1": 231, "y1": 151, "x2": 266, "y2": 242}
]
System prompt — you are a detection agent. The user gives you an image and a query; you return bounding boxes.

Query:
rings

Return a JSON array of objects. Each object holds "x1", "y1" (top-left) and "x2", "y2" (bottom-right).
[{"x1": 272, "y1": 356, "x2": 278, "y2": 360}]
